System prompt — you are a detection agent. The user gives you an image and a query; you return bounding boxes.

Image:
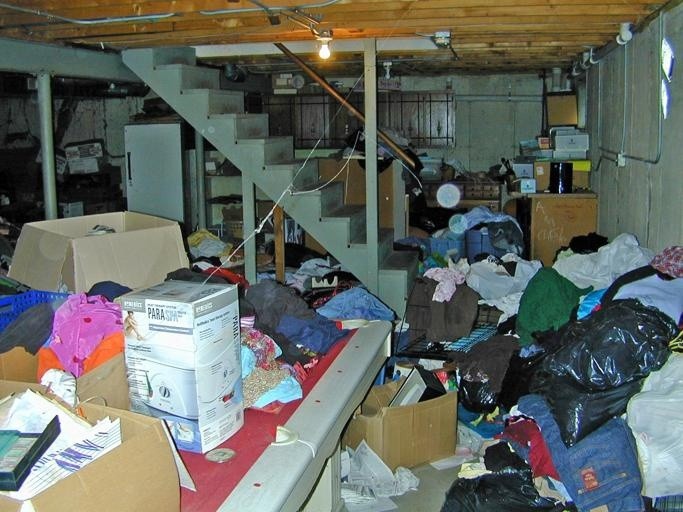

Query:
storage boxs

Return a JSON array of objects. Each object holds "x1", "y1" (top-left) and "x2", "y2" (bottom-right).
[
  {"x1": 6, "y1": 211, "x2": 189, "y2": 294},
  {"x1": 546, "y1": 94, "x2": 578, "y2": 126},
  {"x1": 550, "y1": 162, "x2": 573, "y2": 194},
  {"x1": 466, "y1": 231, "x2": 504, "y2": 265},
  {"x1": 430, "y1": 239, "x2": 465, "y2": 262},
  {"x1": 513, "y1": 161, "x2": 534, "y2": 178},
  {"x1": 538, "y1": 137, "x2": 551, "y2": 149},
  {"x1": 0, "y1": 289, "x2": 70, "y2": 334},
  {"x1": 114, "y1": 278, "x2": 244, "y2": 456},
  {"x1": 533, "y1": 161, "x2": 551, "y2": 192},
  {"x1": 572, "y1": 161, "x2": 591, "y2": 172},
  {"x1": 553, "y1": 133, "x2": 589, "y2": 152},
  {"x1": 542, "y1": 150, "x2": 553, "y2": 158},
  {"x1": 0, "y1": 401, "x2": 181, "y2": 503},
  {"x1": 520, "y1": 179, "x2": 537, "y2": 193},
  {"x1": 1, "y1": 345, "x2": 129, "y2": 409},
  {"x1": 1, "y1": 353, "x2": 131, "y2": 412},
  {"x1": 317, "y1": 159, "x2": 394, "y2": 229},
  {"x1": 554, "y1": 152, "x2": 587, "y2": 160},
  {"x1": 572, "y1": 172, "x2": 590, "y2": 191}
]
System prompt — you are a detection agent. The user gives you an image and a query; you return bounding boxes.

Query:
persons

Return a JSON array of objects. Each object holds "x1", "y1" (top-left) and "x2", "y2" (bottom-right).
[{"x1": 122, "y1": 311, "x2": 146, "y2": 341}]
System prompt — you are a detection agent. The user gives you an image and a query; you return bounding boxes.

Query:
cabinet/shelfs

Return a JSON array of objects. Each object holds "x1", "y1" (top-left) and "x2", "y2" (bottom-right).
[
  {"x1": 423, "y1": 181, "x2": 499, "y2": 215},
  {"x1": 124, "y1": 116, "x2": 184, "y2": 223}
]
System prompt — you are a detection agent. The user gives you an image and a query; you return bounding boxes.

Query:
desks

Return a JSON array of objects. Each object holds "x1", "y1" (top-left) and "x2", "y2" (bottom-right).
[
  {"x1": 527, "y1": 193, "x2": 598, "y2": 269},
  {"x1": 180, "y1": 320, "x2": 393, "y2": 502}
]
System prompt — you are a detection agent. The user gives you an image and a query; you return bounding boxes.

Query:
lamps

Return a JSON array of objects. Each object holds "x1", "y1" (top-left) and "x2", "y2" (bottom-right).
[{"x1": 315, "y1": 29, "x2": 333, "y2": 60}]
